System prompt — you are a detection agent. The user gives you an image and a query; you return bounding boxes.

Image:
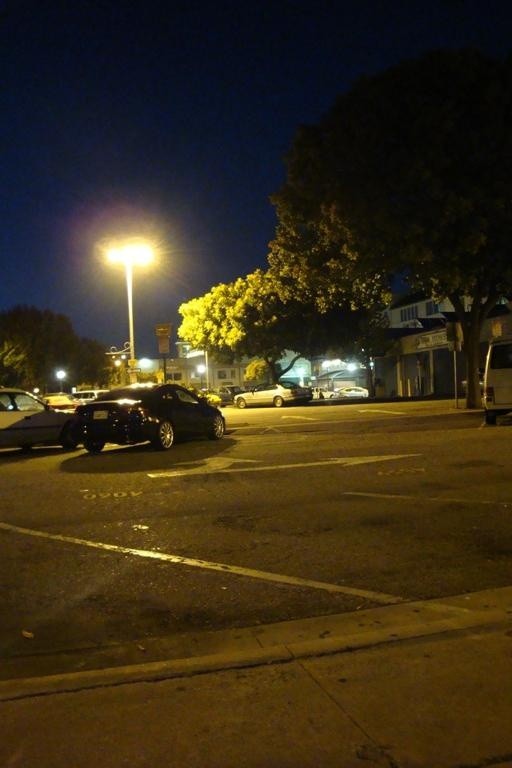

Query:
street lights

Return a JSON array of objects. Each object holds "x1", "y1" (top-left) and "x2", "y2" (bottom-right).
[{"x1": 110, "y1": 243, "x2": 153, "y2": 384}]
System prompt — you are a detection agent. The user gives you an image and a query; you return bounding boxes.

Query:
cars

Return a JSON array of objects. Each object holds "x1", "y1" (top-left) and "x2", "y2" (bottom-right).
[
  {"x1": 313, "y1": 387, "x2": 334, "y2": 399},
  {"x1": 233, "y1": 381, "x2": 313, "y2": 408},
  {"x1": 74, "y1": 383, "x2": 225, "y2": 454},
  {"x1": 220, "y1": 386, "x2": 244, "y2": 407},
  {"x1": 0, "y1": 388, "x2": 109, "y2": 450},
  {"x1": 338, "y1": 387, "x2": 368, "y2": 399}
]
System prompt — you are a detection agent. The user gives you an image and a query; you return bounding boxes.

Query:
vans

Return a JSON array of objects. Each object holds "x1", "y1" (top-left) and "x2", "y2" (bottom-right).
[{"x1": 483, "y1": 336, "x2": 512, "y2": 423}]
